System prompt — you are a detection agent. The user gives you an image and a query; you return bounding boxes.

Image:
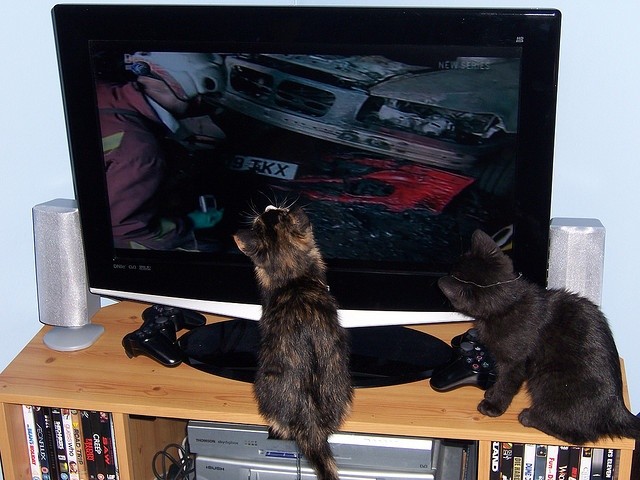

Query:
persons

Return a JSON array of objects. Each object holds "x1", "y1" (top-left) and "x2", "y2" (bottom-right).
[{"x1": 87, "y1": 38, "x2": 223, "y2": 255}]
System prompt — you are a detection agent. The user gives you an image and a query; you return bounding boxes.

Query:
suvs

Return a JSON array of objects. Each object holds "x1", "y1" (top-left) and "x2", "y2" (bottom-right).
[{"x1": 187, "y1": 50, "x2": 516, "y2": 264}]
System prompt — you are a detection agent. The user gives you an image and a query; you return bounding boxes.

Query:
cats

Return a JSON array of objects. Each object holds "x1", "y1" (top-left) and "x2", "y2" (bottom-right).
[
  {"x1": 232, "y1": 193, "x2": 356, "y2": 480},
  {"x1": 436, "y1": 229, "x2": 640, "y2": 446}
]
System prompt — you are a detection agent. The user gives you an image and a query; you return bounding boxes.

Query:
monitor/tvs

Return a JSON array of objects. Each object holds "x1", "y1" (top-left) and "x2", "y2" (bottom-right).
[{"x1": 50, "y1": 4, "x2": 563, "y2": 389}]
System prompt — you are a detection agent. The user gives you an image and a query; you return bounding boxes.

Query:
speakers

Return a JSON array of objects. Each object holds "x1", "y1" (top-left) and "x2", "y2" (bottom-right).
[
  {"x1": 546, "y1": 217, "x2": 606, "y2": 312},
  {"x1": 32, "y1": 197, "x2": 106, "y2": 352}
]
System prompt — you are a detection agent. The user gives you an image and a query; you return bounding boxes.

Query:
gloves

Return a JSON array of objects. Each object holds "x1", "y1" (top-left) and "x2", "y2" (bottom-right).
[{"x1": 190, "y1": 209, "x2": 224, "y2": 229}]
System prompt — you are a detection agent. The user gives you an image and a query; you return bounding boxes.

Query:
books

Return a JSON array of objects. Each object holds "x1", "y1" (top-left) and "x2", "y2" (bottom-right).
[
  {"x1": 18, "y1": 403, "x2": 118, "y2": 479},
  {"x1": 491, "y1": 440, "x2": 623, "y2": 480}
]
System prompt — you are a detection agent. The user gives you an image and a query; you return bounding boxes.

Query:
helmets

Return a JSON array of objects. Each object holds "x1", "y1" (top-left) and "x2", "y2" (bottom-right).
[{"x1": 125, "y1": 51, "x2": 229, "y2": 102}]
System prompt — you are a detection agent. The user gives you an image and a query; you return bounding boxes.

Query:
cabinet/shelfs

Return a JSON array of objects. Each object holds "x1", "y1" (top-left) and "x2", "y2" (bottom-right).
[{"x1": 0, "y1": 301, "x2": 635, "y2": 480}]
